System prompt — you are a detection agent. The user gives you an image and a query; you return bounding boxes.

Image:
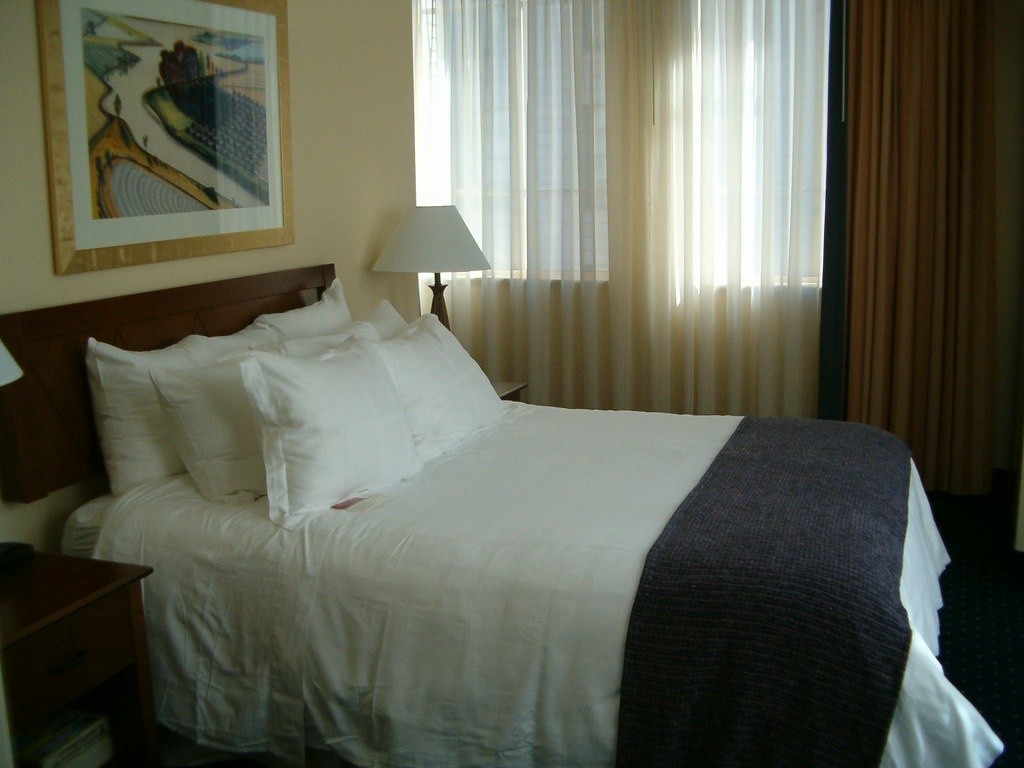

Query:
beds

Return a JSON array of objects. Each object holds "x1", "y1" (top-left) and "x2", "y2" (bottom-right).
[{"x1": 0, "y1": 258, "x2": 1009, "y2": 768}]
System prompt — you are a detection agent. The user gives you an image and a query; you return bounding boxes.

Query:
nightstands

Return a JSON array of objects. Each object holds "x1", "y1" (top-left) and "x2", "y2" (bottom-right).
[
  {"x1": 0, "y1": 541, "x2": 161, "y2": 768},
  {"x1": 488, "y1": 375, "x2": 530, "y2": 406}
]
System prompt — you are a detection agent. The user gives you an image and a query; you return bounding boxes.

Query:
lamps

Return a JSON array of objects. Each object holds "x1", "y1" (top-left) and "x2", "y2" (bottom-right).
[
  {"x1": 0, "y1": 335, "x2": 32, "y2": 392},
  {"x1": 368, "y1": 202, "x2": 495, "y2": 338}
]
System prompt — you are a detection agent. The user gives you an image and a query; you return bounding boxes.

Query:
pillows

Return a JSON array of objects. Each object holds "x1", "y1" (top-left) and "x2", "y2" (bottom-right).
[{"x1": 78, "y1": 276, "x2": 512, "y2": 531}]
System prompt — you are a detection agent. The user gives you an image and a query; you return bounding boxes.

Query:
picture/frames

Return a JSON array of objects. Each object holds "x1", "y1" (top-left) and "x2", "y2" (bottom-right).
[{"x1": 33, "y1": 0, "x2": 298, "y2": 279}]
